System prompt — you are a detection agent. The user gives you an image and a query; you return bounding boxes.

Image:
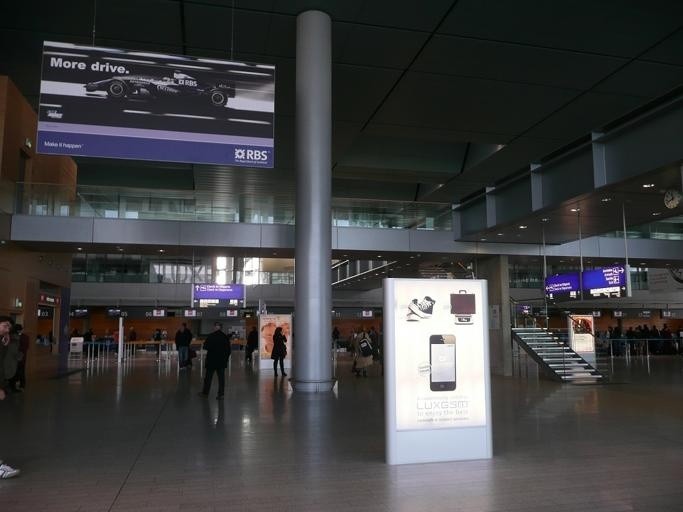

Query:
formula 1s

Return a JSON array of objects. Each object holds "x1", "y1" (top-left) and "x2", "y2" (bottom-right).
[{"x1": 85, "y1": 69, "x2": 235, "y2": 108}]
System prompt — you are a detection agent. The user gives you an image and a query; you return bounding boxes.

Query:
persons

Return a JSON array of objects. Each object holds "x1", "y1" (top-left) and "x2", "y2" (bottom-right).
[
  {"x1": 197, "y1": 321, "x2": 232, "y2": 400},
  {"x1": 175, "y1": 323, "x2": 192, "y2": 370},
  {"x1": 152, "y1": 328, "x2": 161, "y2": 355},
  {"x1": 332, "y1": 325, "x2": 378, "y2": 377},
  {"x1": 245, "y1": 326, "x2": 258, "y2": 362},
  {"x1": 70, "y1": 325, "x2": 136, "y2": 359},
  {"x1": 0, "y1": 315, "x2": 29, "y2": 479},
  {"x1": 271, "y1": 327, "x2": 287, "y2": 377},
  {"x1": 594, "y1": 323, "x2": 672, "y2": 357}
]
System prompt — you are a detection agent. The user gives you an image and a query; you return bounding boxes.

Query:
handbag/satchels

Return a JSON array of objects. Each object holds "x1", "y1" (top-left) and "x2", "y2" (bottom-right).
[{"x1": 359, "y1": 338, "x2": 373, "y2": 357}]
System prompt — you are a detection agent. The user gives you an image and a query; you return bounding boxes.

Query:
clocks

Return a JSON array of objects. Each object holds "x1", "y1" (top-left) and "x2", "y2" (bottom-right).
[{"x1": 662, "y1": 187, "x2": 682, "y2": 210}]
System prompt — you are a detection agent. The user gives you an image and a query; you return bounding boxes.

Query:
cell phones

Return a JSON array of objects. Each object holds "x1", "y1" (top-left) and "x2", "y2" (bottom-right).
[{"x1": 429, "y1": 335, "x2": 456, "y2": 391}]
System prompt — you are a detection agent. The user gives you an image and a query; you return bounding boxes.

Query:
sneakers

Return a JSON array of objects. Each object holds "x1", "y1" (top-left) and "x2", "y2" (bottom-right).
[
  {"x1": 198, "y1": 392, "x2": 223, "y2": 400},
  {"x1": 0, "y1": 464, "x2": 20, "y2": 479},
  {"x1": 406, "y1": 296, "x2": 435, "y2": 321}
]
